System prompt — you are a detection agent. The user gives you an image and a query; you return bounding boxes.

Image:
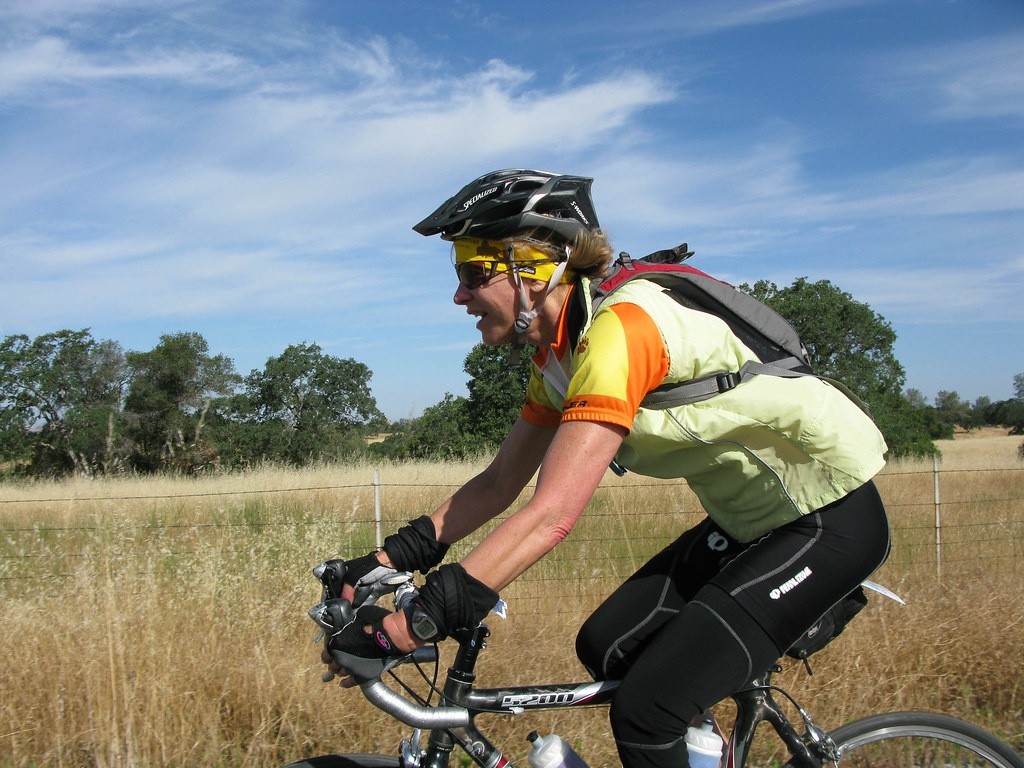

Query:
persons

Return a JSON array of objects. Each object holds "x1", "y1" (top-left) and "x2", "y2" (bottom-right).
[{"x1": 321, "y1": 168, "x2": 891, "y2": 768}]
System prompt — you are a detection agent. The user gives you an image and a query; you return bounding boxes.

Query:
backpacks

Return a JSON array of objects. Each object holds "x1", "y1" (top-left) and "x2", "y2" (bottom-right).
[{"x1": 579, "y1": 242, "x2": 815, "y2": 409}]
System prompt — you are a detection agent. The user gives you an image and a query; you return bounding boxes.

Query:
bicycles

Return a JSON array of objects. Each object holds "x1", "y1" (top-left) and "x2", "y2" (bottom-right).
[{"x1": 277, "y1": 558, "x2": 1024, "y2": 768}]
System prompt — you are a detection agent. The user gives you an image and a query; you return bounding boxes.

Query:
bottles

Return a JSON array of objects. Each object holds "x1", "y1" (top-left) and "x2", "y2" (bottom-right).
[
  {"x1": 527, "y1": 730, "x2": 589, "y2": 768},
  {"x1": 683, "y1": 718, "x2": 723, "y2": 768}
]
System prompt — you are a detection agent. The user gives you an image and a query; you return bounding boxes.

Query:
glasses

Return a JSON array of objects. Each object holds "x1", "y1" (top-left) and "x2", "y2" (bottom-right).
[{"x1": 456, "y1": 255, "x2": 566, "y2": 293}]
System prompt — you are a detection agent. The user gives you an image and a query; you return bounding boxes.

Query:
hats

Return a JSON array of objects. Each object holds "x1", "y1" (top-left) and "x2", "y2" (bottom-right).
[{"x1": 452, "y1": 233, "x2": 577, "y2": 286}]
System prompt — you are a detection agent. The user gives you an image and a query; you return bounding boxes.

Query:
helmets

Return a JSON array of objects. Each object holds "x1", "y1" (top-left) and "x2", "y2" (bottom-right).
[{"x1": 412, "y1": 168, "x2": 603, "y2": 244}]
